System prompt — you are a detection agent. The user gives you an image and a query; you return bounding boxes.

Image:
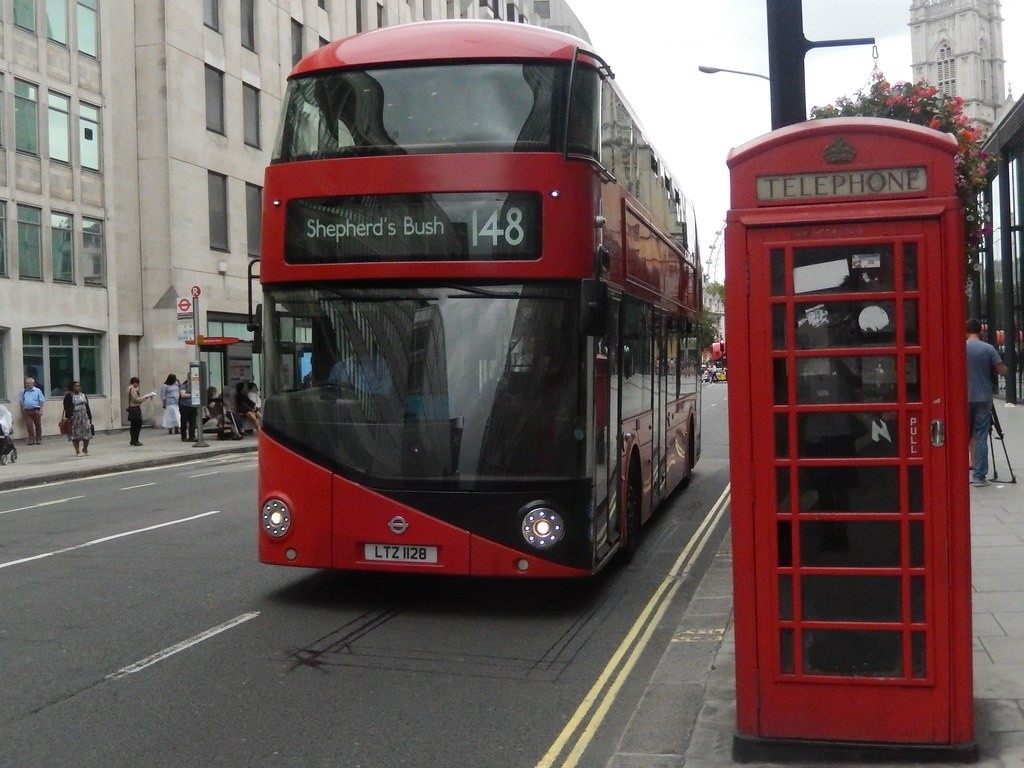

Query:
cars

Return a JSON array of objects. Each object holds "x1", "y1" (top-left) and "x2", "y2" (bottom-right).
[{"x1": 702, "y1": 368, "x2": 726, "y2": 382}]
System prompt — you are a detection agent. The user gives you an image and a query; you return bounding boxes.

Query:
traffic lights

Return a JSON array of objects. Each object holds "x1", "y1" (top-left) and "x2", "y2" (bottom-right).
[{"x1": 720, "y1": 338, "x2": 724, "y2": 352}]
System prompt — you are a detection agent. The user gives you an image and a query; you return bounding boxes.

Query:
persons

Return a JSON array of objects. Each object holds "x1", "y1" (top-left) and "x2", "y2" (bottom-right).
[
  {"x1": 964, "y1": 320, "x2": 1010, "y2": 487},
  {"x1": 61, "y1": 381, "x2": 93, "y2": 456},
  {"x1": 326, "y1": 330, "x2": 395, "y2": 402},
  {"x1": 653, "y1": 355, "x2": 697, "y2": 379},
  {"x1": 202, "y1": 382, "x2": 261, "y2": 440},
  {"x1": 804, "y1": 310, "x2": 880, "y2": 557},
  {"x1": 20, "y1": 377, "x2": 45, "y2": 445},
  {"x1": 701, "y1": 362, "x2": 727, "y2": 383},
  {"x1": 124, "y1": 376, "x2": 150, "y2": 446},
  {"x1": 160, "y1": 374, "x2": 181, "y2": 435},
  {"x1": 178, "y1": 371, "x2": 199, "y2": 442}
]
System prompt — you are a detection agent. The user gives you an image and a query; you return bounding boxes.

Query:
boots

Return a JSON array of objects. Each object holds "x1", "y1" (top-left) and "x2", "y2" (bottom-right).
[
  {"x1": 169, "y1": 428, "x2": 172, "y2": 434},
  {"x1": 175, "y1": 426, "x2": 180, "y2": 434}
]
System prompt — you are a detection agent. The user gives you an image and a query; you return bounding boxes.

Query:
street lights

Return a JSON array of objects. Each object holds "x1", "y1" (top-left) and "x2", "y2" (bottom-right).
[{"x1": 699, "y1": 66, "x2": 770, "y2": 80}]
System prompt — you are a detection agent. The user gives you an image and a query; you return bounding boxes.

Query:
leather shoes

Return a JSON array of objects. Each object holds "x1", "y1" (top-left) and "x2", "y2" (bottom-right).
[{"x1": 130, "y1": 441, "x2": 143, "y2": 446}]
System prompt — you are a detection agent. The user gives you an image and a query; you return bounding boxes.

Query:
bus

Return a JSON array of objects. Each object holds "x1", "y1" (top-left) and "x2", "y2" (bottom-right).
[{"x1": 249, "y1": 20, "x2": 702, "y2": 576}]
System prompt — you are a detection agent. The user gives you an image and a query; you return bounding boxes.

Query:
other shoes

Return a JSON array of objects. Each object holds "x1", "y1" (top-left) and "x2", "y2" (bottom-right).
[
  {"x1": 182, "y1": 437, "x2": 188, "y2": 442},
  {"x1": 36, "y1": 439, "x2": 42, "y2": 444},
  {"x1": 189, "y1": 438, "x2": 199, "y2": 442},
  {"x1": 28, "y1": 439, "x2": 35, "y2": 444},
  {"x1": 239, "y1": 432, "x2": 247, "y2": 436},
  {"x1": 75, "y1": 452, "x2": 85, "y2": 457},
  {"x1": 81, "y1": 449, "x2": 91, "y2": 456}
]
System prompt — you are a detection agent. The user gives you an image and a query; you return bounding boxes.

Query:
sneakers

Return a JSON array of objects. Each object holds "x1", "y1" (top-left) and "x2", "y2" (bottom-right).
[{"x1": 973, "y1": 479, "x2": 991, "y2": 487}]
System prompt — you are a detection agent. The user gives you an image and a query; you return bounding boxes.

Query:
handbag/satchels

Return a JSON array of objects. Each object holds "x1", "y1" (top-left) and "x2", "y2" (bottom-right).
[
  {"x1": 59, "y1": 420, "x2": 72, "y2": 433},
  {"x1": 163, "y1": 399, "x2": 166, "y2": 409}
]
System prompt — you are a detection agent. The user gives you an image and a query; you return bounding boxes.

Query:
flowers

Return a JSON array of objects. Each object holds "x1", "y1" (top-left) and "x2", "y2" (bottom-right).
[{"x1": 808, "y1": 80, "x2": 1004, "y2": 256}]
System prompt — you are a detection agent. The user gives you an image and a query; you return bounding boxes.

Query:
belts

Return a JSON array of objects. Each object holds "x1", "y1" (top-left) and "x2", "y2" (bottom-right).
[{"x1": 25, "y1": 408, "x2": 40, "y2": 411}]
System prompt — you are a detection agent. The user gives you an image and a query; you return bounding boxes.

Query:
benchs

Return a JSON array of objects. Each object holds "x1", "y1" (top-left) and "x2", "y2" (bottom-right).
[{"x1": 200, "y1": 409, "x2": 260, "y2": 440}]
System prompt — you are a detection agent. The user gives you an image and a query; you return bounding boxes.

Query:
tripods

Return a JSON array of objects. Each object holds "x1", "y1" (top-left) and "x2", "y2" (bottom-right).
[{"x1": 988, "y1": 403, "x2": 1017, "y2": 484}]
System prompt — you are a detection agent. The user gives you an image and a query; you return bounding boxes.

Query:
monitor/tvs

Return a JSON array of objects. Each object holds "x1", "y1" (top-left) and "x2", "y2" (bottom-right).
[{"x1": 859, "y1": 333, "x2": 920, "y2": 396}]
